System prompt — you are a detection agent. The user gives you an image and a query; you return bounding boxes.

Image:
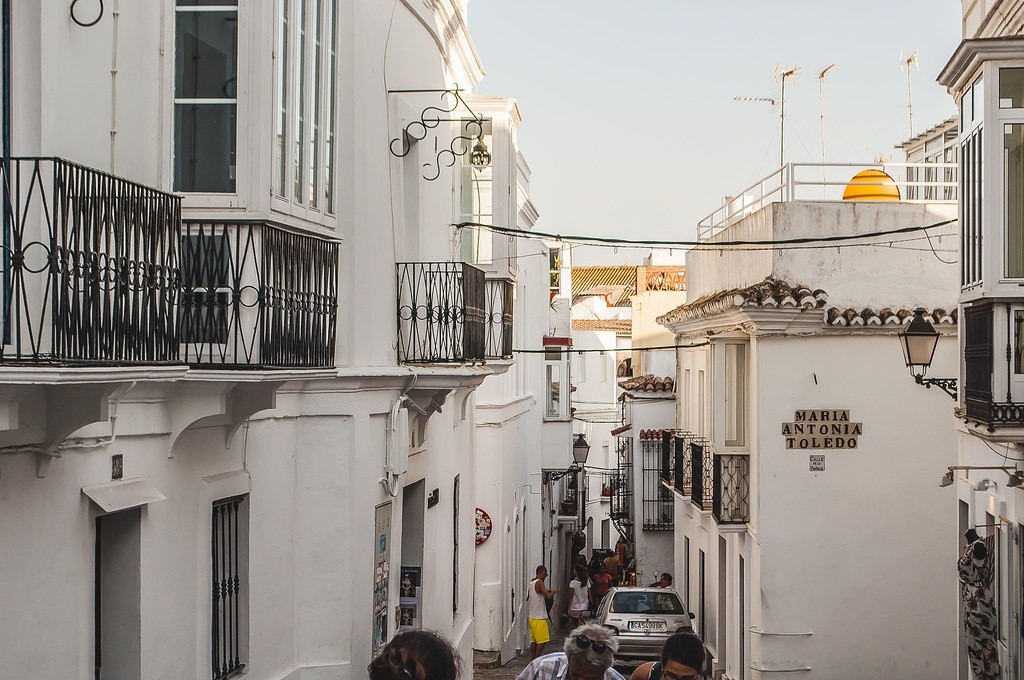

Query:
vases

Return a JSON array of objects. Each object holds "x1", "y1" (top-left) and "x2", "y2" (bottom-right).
[{"x1": 561, "y1": 499, "x2": 577, "y2": 516}]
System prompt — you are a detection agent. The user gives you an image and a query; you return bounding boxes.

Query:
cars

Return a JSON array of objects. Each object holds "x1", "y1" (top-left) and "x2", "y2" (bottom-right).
[{"x1": 578, "y1": 583, "x2": 697, "y2": 662}]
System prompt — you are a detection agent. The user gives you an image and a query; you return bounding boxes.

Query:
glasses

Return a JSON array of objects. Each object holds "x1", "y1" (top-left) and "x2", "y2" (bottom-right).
[
  {"x1": 662, "y1": 668, "x2": 701, "y2": 680},
  {"x1": 572, "y1": 634, "x2": 613, "y2": 654}
]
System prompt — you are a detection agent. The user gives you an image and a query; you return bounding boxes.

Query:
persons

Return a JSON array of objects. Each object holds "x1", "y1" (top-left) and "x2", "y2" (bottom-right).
[
  {"x1": 400, "y1": 574, "x2": 415, "y2": 597},
  {"x1": 369, "y1": 630, "x2": 456, "y2": 680},
  {"x1": 529, "y1": 565, "x2": 558, "y2": 660},
  {"x1": 656, "y1": 573, "x2": 672, "y2": 611},
  {"x1": 569, "y1": 535, "x2": 626, "y2": 630},
  {"x1": 631, "y1": 633, "x2": 706, "y2": 680},
  {"x1": 514, "y1": 624, "x2": 626, "y2": 680},
  {"x1": 401, "y1": 609, "x2": 411, "y2": 625}
]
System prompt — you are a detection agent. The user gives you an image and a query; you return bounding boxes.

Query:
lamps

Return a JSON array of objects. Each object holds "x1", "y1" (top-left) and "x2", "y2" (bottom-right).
[
  {"x1": 470, "y1": 122, "x2": 491, "y2": 173},
  {"x1": 542, "y1": 431, "x2": 591, "y2": 486},
  {"x1": 901, "y1": 305, "x2": 958, "y2": 404},
  {"x1": 939, "y1": 463, "x2": 1024, "y2": 491}
]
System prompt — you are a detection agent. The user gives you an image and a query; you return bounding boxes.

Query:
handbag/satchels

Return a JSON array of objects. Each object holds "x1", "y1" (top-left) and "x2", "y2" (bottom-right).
[{"x1": 545, "y1": 590, "x2": 554, "y2": 615}]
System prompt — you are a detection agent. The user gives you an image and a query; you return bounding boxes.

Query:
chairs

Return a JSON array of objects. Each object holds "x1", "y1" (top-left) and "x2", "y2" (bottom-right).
[{"x1": 615, "y1": 603, "x2": 631, "y2": 613}]
